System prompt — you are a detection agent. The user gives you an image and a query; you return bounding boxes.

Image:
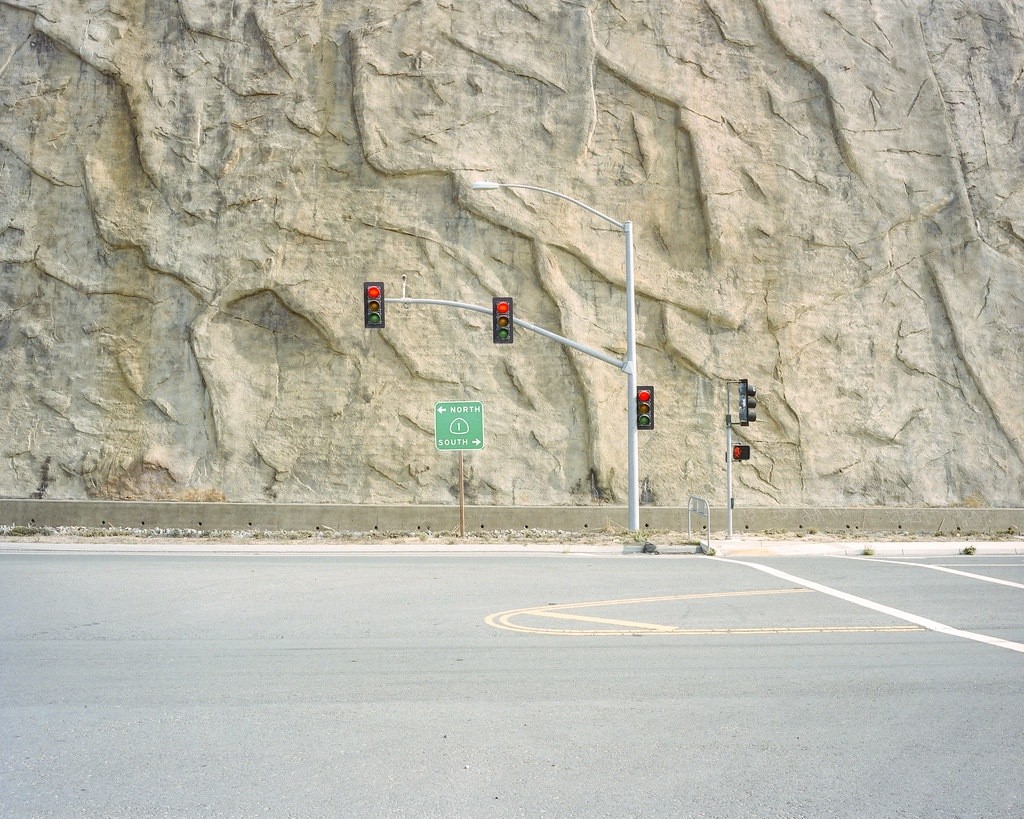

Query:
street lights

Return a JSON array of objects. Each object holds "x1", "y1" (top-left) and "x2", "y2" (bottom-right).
[{"x1": 472, "y1": 183, "x2": 642, "y2": 530}]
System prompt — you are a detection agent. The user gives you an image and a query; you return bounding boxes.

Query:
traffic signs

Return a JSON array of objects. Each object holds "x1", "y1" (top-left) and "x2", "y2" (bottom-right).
[{"x1": 434, "y1": 401, "x2": 484, "y2": 452}]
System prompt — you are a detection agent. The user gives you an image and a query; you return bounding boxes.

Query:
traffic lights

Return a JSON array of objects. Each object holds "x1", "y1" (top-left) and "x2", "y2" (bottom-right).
[
  {"x1": 637, "y1": 385, "x2": 655, "y2": 429},
  {"x1": 491, "y1": 296, "x2": 513, "y2": 345},
  {"x1": 362, "y1": 281, "x2": 385, "y2": 329},
  {"x1": 738, "y1": 377, "x2": 756, "y2": 427},
  {"x1": 733, "y1": 444, "x2": 750, "y2": 460}
]
